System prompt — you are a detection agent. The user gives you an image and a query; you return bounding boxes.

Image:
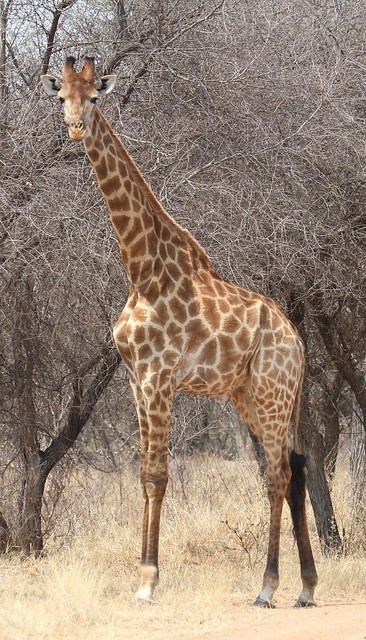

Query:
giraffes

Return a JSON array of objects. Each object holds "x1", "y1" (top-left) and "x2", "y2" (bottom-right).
[{"x1": 38, "y1": 55, "x2": 319, "y2": 609}]
